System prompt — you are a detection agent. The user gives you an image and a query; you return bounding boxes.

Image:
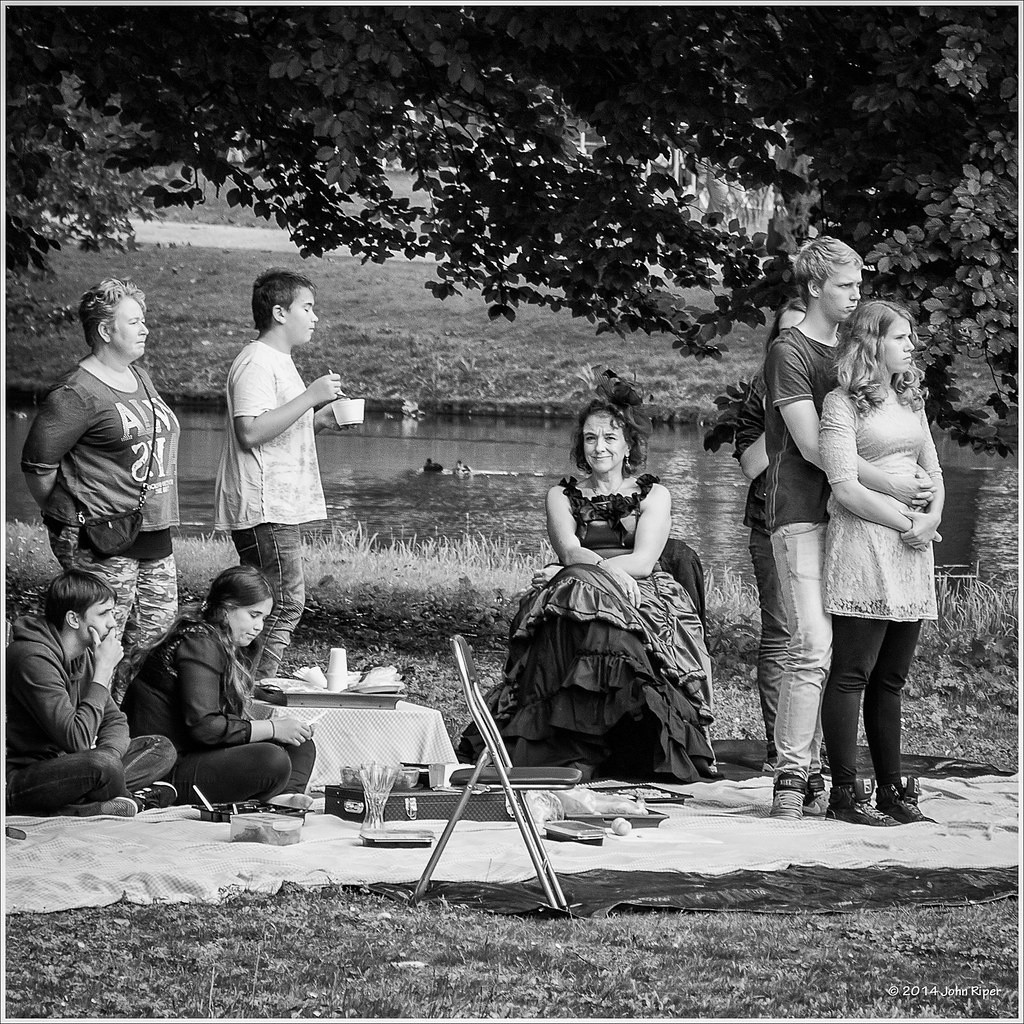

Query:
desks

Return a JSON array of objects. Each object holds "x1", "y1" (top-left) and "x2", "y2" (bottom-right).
[{"x1": 241, "y1": 699, "x2": 459, "y2": 794}]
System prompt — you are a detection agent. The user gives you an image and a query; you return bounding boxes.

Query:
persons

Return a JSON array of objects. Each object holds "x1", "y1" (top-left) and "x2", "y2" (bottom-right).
[
  {"x1": 19, "y1": 276, "x2": 183, "y2": 703},
  {"x1": 6, "y1": 567, "x2": 179, "y2": 818},
  {"x1": 117, "y1": 565, "x2": 318, "y2": 812},
  {"x1": 763, "y1": 235, "x2": 937, "y2": 822},
  {"x1": 424, "y1": 458, "x2": 473, "y2": 478},
  {"x1": 734, "y1": 294, "x2": 833, "y2": 774},
  {"x1": 493, "y1": 394, "x2": 725, "y2": 785},
  {"x1": 211, "y1": 265, "x2": 361, "y2": 681},
  {"x1": 816, "y1": 300, "x2": 948, "y2": 832}
]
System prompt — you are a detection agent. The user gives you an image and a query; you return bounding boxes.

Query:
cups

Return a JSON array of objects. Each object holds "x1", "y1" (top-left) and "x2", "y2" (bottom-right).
[
  {"x1": 358, "y1": 792, "x2": 390, "y2": 831},
  {"x1": 327, "y1": 648, "x2": 347, "y2": 692}
]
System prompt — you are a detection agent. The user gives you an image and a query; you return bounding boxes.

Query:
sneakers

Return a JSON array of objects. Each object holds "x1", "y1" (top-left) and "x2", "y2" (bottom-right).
[
  {"x1": 56, "y1": 797, "x2": 144, "y2": 817},
  {"x1": 876, "y1": 775, "x2": 938, "y2": 824},
  {"x1": 825, "y1": 778, "x2": 902, "y2": 827},
  {"x1": 770, "y1": 772, "x2": 807, "y2": 822},
  {"x1": 130, "y1": 782, "x2": 178, "y2": 810}
]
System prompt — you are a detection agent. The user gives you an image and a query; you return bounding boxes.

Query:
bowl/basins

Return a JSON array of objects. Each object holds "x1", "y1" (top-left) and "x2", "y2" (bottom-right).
[
  {"x1": 332, "y1": 399, "x2": 365, "y2": 426},
  {"x1": 341, "y1": 766, "x2": 419, "y2": 790}
]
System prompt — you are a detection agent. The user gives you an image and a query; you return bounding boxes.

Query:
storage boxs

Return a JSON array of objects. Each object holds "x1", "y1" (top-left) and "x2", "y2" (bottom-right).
[{"x1": 228, "y1": 812, "x2": 304, "y2": 847}]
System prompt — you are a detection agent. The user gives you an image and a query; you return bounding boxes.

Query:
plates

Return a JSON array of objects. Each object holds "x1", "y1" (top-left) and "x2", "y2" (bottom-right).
[{"x1": 347, "y1": 683, "x2": 401, "y2": 693}]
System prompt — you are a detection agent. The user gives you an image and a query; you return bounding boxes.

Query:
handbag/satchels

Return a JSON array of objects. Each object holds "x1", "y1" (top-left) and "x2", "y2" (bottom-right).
[{"x1": 83, "y1": 509, "x2": 143, "y2": 556}]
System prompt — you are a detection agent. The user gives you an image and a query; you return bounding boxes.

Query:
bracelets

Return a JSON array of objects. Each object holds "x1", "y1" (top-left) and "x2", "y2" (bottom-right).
[
  {"x1": 269, "y1": 719, "x2": 275, "y2": 741},
  {"x1": 596, "y1": 558, "x2": 607, "y2": 568}
]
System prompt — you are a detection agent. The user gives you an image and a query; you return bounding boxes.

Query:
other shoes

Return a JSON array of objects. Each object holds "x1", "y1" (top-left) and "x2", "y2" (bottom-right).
[
  {"x1": 569, "y1": 762, "x2": 599, "y2": 783},
  {"x1": 802, "y1": 774, "x2": 829, "y2": 816}
]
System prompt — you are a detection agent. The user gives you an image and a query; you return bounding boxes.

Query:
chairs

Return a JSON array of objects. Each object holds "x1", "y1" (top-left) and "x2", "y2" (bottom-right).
[
  {"x1": 408, "y1": 635, "x2": 583, "y2": 909},
  {"x1": 658, "y1": 538, "x2": 709, "y2": 649}
]
